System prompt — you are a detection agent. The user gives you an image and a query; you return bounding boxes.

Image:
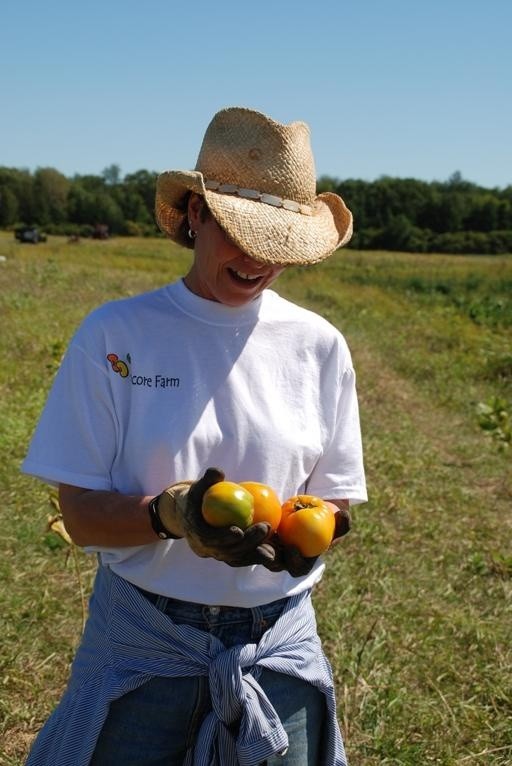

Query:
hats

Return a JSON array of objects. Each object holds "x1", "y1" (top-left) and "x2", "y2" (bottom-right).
[{"x1": 156, "y1": 106, "x2": 353, "y2": 267}]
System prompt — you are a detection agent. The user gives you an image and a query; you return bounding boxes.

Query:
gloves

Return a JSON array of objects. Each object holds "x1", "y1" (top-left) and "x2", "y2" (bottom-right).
[
  {"x1": 256, "y1": 507, "x2": 352, "y2": 577},
  {"x1": 160, "y1": 465, "x2": 274, "y2": 567}
]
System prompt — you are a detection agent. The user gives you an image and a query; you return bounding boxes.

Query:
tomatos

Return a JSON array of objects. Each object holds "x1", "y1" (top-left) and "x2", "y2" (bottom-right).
[
  {"x1": 201, "y1": 481, "x2": 255, "y2": 532},
  {"x1": 238, "y1": 481, "x2": 282, "y2": 537},
  {"x1": 277, "y1": 494, "x2": 337, "y2": 559}
]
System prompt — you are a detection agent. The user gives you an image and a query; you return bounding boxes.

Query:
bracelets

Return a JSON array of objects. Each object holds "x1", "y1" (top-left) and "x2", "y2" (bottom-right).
[{"x1": 147, "y1": 494, "x2": 183, "y2": 541}]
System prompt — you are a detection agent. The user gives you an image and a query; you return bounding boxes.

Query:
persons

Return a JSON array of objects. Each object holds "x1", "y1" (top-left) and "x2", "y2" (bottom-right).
[{"x1": 18, "y1": 104, "x2": 372, "y2": 764}]
